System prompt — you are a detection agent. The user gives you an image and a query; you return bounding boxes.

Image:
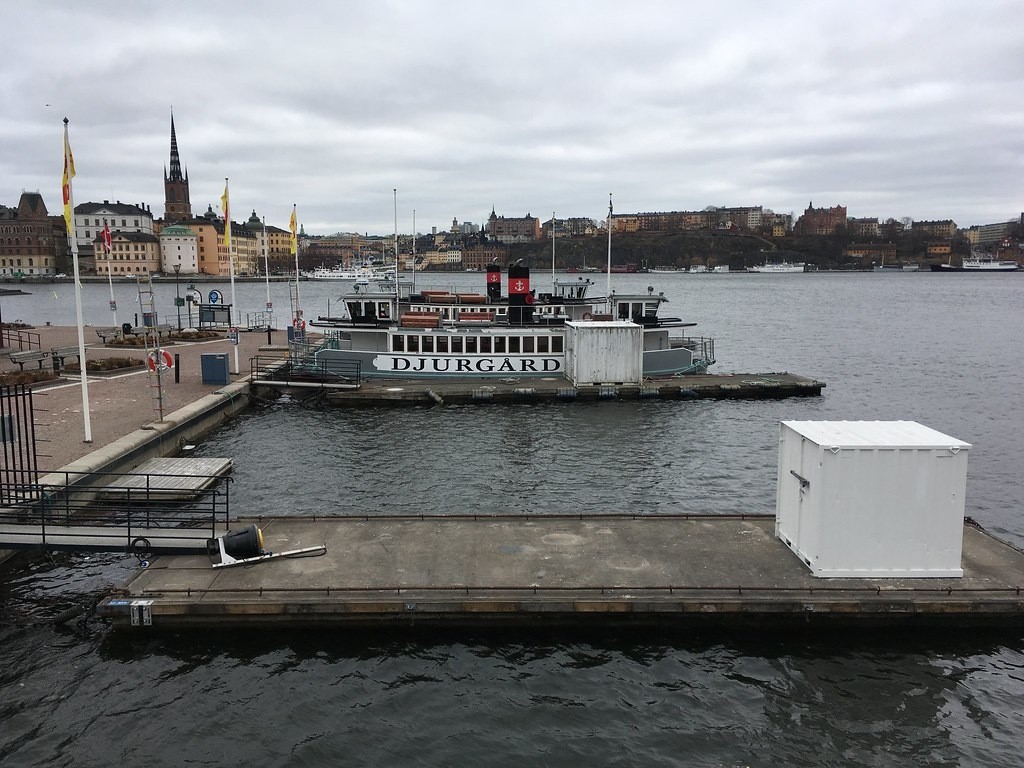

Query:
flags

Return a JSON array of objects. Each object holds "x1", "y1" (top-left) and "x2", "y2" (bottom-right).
[
  {"x1": 289, "y1": 210, "x2": 296, "y2": 255},
  {"x1": 607, "y1": 200, "x2": 614, "y2": 218},
  {"x1": 221, "y1": 184, "x2": 231, "y2": 247},
  {"x1": 62, "y1": 126, "x2": 77, "y2": 237},
  {"x1": 552, "y1": 217, "x2": 557, "y2": 232},
  {"x1": 100, "y1": 221, "x2": 111, "y2": 253}
]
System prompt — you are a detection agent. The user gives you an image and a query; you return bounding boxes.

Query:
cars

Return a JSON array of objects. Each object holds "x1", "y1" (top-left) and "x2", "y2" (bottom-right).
[
  {"x1": 126, "y1": 273, "x2": 135, "y2": 279},
  {"x1": 55, "y1": 273, "x2": 67, "y2": 278},
  {"x1": 152, "y1": 274, "x2": 160, "y2": 279}
]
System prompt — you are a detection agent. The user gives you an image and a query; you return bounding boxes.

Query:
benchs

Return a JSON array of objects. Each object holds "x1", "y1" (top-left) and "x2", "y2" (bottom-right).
[
  {"x1": 10, "y1": 350, "x2": 49, "y2": 371},
  {"x1": 130, "y1": 327, "x2": 151, "y2": 337},
  {"x1": 156, "y1": 324, "x2": 172, "y2": 338},
  {"x1": 96, "y1": 329, "x2": 123, "y2": 344},
  {"x1": 51, "y1": 347, "x2": 88, "y2": 366}
]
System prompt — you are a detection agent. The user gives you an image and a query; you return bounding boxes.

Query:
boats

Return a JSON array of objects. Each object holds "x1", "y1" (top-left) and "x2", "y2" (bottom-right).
[
  {"x1": 309, "y1": 188, "x2": 716, "y2": 379},
  {"x1": 873, "y1": 253, "x2": 920, "y2": 272},
  {"x1": 930, "y1": 254, "x2": 1019, "y2": 272},
  {"x1": 747, "y1": 259, "x2": 805, "y2": 273}
]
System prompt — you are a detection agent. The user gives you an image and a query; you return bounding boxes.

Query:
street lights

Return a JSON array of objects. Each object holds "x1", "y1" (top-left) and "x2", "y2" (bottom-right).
[{"x1": 172, "y1": 264, "x2": 181, "y2": 333}]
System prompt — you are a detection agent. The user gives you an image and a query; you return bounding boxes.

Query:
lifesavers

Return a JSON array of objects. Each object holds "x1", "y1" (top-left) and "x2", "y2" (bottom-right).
[
  {"x1": 292, "y1": 317, "x2": 305, "y2": 330},
  {"x1": 148, "y1": 349, "x2": 172, "y2": 374}
]
